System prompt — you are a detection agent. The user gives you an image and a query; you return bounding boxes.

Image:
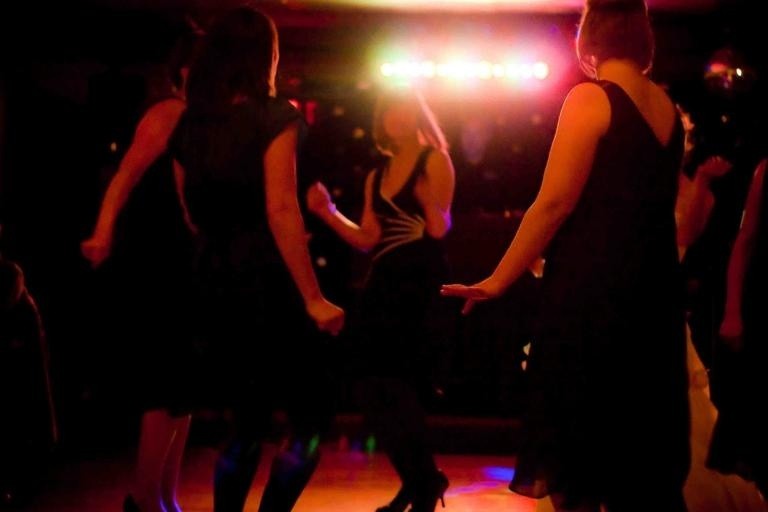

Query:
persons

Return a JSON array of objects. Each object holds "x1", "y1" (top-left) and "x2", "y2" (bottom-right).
[
  {"x1": 667, "y1": 98, "x2": 733, "y2": 263},
  {"x1": 436, "y1": 0, "x2": 694, "y2": 511},
  {"x1": 303, "y1": 85, "x2": 459, "y2": 512},
  {"x1": 451, "y1": 105, "x2": 526, "y2": 216},
  {"x1": 77, "y1": 8, "x2": 200, "y2": 512},
  {"x1": 163, "y1": 3, "x2": 348, "y2": 511},
  {"x1": 715, "y1": 156, "x2": 768, "y2": 501},
  {"x1": 0, "y1": 258, "x2": 63, "y2": 512}
]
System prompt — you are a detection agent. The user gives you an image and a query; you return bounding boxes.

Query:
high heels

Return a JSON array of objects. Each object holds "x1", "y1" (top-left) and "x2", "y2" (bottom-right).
[{"x1": 409, "y1": 470, "x2": 448, "y2": 512}]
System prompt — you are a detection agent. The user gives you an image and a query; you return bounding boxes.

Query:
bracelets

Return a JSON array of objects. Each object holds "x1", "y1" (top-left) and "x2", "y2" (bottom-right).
[{"x1": 316, "y1": 202, "x2": 337, "y2": 223}]
